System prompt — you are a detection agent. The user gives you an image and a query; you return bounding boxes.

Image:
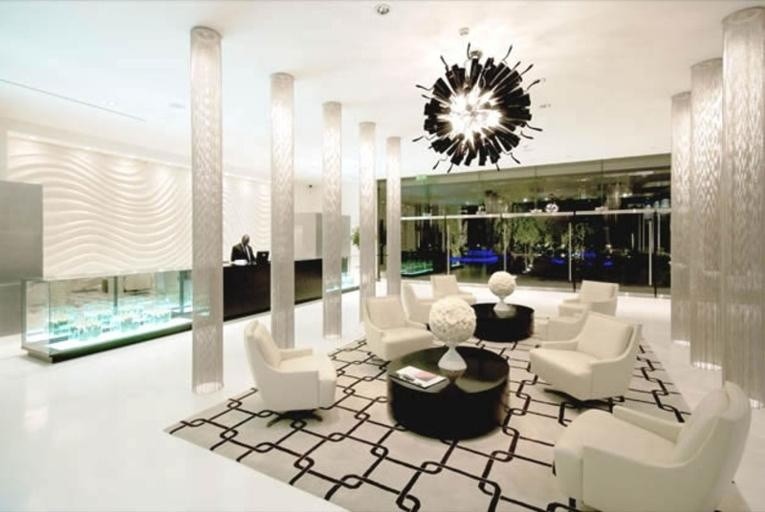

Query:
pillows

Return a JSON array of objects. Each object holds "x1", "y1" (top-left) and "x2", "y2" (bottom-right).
[{"x1": 253, "y1": 321, "x2": 281, "y2": 368}]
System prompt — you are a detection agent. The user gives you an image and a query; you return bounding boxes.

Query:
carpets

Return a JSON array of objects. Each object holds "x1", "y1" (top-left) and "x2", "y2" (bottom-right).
[{"x1": 164, "y1": 317, "x2": 750, "y2": 512}]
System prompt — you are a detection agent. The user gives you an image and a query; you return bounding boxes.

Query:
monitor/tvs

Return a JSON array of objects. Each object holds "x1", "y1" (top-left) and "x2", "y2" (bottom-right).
[{"x1": 256, "y1": 251, "x2": 269, "y2": 264}]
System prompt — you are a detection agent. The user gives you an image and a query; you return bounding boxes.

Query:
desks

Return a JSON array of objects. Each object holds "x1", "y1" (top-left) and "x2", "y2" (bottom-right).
[
  {"x1": 387, "y1": 346, "x2": 510, "y2": 439},
  {"x1": 471, "y1": 303, "x2": 535, "y2": 342}
]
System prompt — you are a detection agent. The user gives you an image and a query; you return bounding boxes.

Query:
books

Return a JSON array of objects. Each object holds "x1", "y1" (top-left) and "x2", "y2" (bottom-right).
[{"x1": 388, "y1": 365, "x2": 446, "y2": 389}]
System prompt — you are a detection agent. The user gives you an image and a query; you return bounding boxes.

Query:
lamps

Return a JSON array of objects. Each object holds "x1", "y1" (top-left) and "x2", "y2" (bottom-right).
[{"x1": 412, "y1": 43, "x2": 550, "y2": 176}]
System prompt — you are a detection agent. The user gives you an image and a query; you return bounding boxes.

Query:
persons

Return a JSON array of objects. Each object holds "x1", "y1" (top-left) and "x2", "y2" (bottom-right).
[{"x1": 231, "y1": 234, "x2": 255, "y2": 261}]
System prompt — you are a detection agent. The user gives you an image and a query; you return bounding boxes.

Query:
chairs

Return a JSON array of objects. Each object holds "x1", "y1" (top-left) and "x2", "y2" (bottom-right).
[
  {"x1": 360, "y1": 274, "x2": 476, "y2": 369},
  {"x1": 244, "y1": 319, "x2": 337, "y2": 422}
]
